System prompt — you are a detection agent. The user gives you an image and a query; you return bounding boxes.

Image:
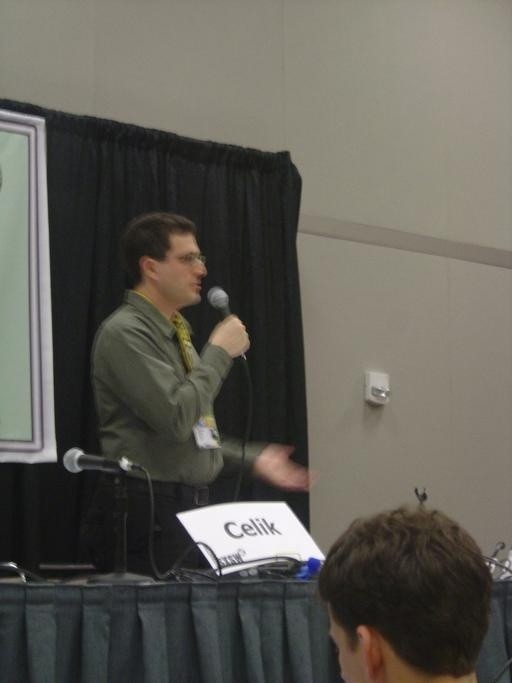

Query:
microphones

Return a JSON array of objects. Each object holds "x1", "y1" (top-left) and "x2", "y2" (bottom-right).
[
  {"x1": 61, "y1": 443, "x2": 134, "y2": 474},
  {"x1": 206, "y1": 286, "x2": 252, "y2": 362}
]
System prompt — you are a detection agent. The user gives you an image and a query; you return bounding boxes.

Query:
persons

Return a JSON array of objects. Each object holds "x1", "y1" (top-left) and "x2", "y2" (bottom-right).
[
  {"x1": 315, "y1": 501, "x2": 494, "y2": 683},
  {"x1": 89, "y1": 210, "x2": 318, "y2": 581}
]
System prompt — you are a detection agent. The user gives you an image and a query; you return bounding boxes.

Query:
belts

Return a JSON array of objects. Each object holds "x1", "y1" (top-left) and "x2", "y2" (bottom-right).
[{"x1": 142, "y1": 479, "x2": 207, "y2": 506}]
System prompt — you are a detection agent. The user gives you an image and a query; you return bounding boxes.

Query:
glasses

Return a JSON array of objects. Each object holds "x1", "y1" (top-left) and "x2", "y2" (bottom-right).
[{"x1": 177, "y1": 254, "x2": 206, "y2": 266}]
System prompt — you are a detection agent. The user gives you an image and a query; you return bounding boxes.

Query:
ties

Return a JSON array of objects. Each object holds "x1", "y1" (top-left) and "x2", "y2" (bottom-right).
[{"x1": 175, "y1": 315, "x2": 216, "y2": 429}]
